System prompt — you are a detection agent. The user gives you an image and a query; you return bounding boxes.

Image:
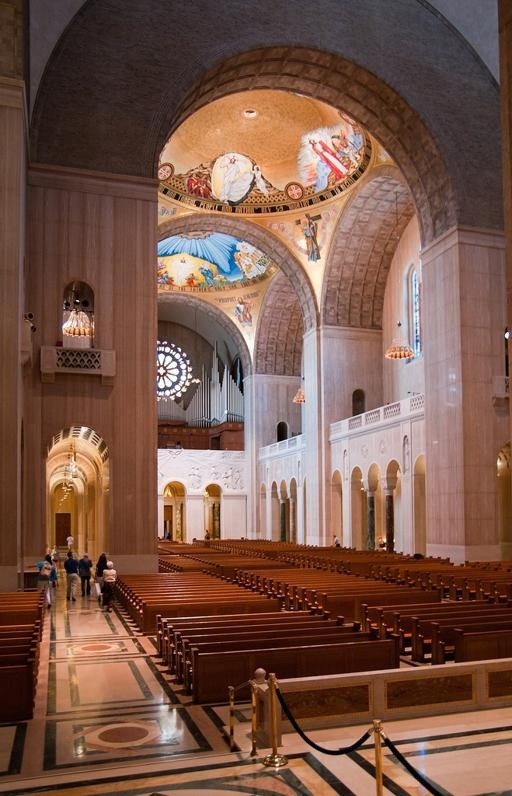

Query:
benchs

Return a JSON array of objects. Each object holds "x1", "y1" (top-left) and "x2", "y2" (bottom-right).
[
  {"x1": 112, "y1": 537, "x2": 512, "y2": 706},
  {"x1": 0, "y1": 584, "x2": 47, "y2": 722}
]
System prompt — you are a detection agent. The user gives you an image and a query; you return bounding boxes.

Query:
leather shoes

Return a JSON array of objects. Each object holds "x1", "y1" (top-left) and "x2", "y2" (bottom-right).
[{"x1": 66, "y1": 596, "x2": 75, "y2": 601}]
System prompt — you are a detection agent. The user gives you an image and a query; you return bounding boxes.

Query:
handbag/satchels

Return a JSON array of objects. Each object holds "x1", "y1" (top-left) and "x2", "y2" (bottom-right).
[{"x1": 94, "y1": 583, "x2": 101, "y2": 597}]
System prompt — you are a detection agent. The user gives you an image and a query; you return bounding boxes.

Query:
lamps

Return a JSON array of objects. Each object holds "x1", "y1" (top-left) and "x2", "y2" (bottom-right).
[
  {"x1": 63, "y1": 442, "x2": 75, "y2": 493},
  {"x1": 204, "y1": 490, "x2": 209, "y2": 498},
  {"x1": 293, "y1": 377, "x2": 306, "y2": 403},
  {"x1": 384, "y1": 320, "x2": 415, "y2": 360},
  {"x1": 164, "y1": 485, "x2": 173, "y2": 498},
  {"x1": 61, "y1": 281, "x2": 92, "y2": 337}
]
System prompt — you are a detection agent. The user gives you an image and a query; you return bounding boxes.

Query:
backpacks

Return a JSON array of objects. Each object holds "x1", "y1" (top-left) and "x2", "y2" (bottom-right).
[{"x1": 40, "y1": 561, "x2": 56, "y2": 578}]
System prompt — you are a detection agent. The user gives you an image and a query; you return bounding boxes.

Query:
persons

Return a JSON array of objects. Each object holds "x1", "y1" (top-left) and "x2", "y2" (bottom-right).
[
  {"x1": 217, "y1": 156, "x2": 249, "y2": 205},
  {"x1": 64, "y1": 551, "x2": 79, "y2": 601},
  {"x1": 307, "y1": 137, "x2": 351, "y2": 182},
  {"x1": 61, "y1": 305, "x2": 92, "y2": 330},
  {"x1": 184, "y1": 273, "x2": 199, "y2": 288},
  {"x1": 300, "y1": 212, "x2": 321, "y2": 264},
  {"x1": 166, "y1": 532, "x2": 172, "y2": 539},
  {"x1": 338, "y1": 111, "x2": 367, "y2": 158},
  {"x1": 204, "y1": 530, "x2": 211, "y2": 540},
  {"x1": 233, "y1": 296, "x2": 255, "y2": 328},
  {"x1": 101, "y1": 560, "x2": 118, "y2": 613},
  {"x1": 78, "y1": 553, "x2": 94, "y2": 597},
  {"x1": 181, "y1": 160, "x2": 214, "y2": 192},
  {"x1": 48, "y1": 544, "x2": 60, "y2": 590},
  {"x1": 328, "y1": 128, "x2": 361, "y2": 169},
  {"x1": 200, "y1": 264, "x2": 215, "y2": 287},
  {"x1": 36, "y1": 554, "x2": 52, "y2": 610},
  {"x1": 188, "y1": 172, "x2": 218, "y2": 202},
  {"x1": 94, "y1": 553, "x2": 110, "y2": 603},
  {"x1": 249, "y1": 164, "x2": 271, "y2": 199},
  {"x1": 67, "y1": 534, "x2": 74, "y2": 551},
  {"x1": 332, "y1": 534, "x2": 341, "y2": 548}
]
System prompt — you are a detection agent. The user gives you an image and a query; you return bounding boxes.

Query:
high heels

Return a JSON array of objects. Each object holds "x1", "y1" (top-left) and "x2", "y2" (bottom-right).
[{"x1": 47, "y1": 603, "x2": 51, "y2": 609}]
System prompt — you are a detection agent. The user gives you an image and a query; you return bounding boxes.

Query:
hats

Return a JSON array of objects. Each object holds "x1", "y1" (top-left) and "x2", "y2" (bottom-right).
[{"x1": 106, "y1": 560, "x2": 114, "y2": 569}]
